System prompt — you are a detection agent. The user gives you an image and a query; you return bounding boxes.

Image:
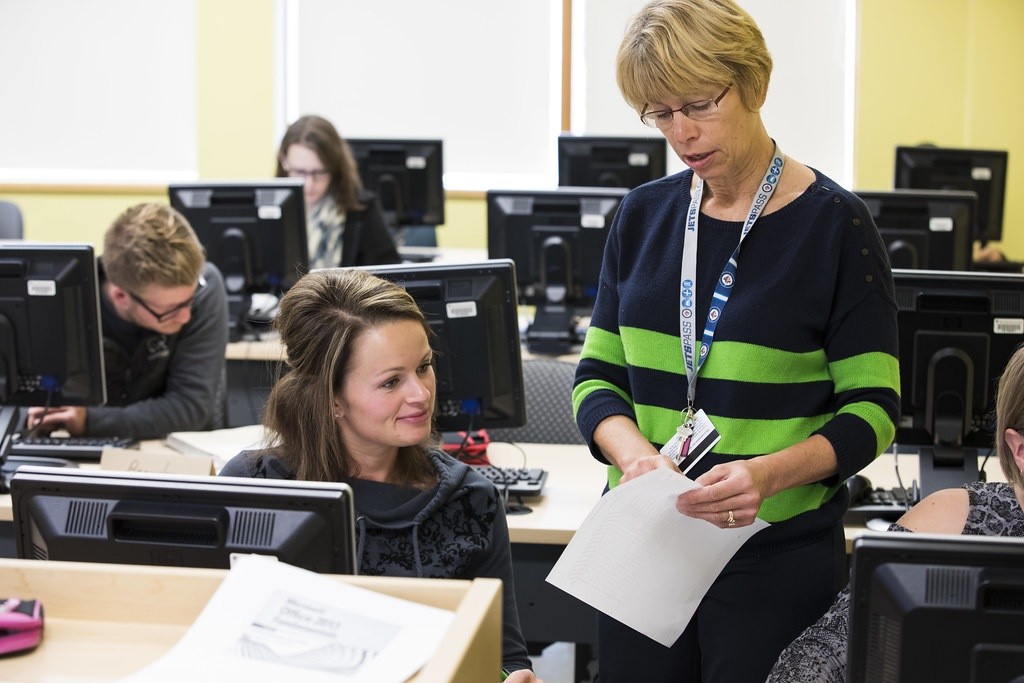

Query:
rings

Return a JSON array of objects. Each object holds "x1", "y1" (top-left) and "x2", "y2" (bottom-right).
[{"x1": 726, "y1": 510, "x2": 736, "y2": 524}]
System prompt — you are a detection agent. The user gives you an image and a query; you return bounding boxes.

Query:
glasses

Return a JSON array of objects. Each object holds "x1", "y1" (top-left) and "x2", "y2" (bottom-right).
[
  {"x1": 126, "y1": 274, "x2": 207, "y2": 322},
  {"x1": 285, "y1": 159, "x2": 331, "y2": 181},
  {"x1": 639, "y1": 77, "x2": 736, "y2": 129}
]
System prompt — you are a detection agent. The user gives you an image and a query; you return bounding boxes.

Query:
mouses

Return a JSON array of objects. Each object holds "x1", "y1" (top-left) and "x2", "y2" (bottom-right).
[{"x1": 844, "y1": 475, "x2": 872, "y2": 501}]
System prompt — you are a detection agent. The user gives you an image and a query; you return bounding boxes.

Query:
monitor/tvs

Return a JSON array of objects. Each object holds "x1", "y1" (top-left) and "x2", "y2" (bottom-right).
[{"x1": 0, "y1": 140, "x2": 1024, "y2": 683}]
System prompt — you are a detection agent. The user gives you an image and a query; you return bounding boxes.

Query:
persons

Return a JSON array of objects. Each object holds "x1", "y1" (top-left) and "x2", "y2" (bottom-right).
[
  {"x1": 915, "y1": 142, "x2": 1009, "y2": 262},
  {"x1": 217, "y1": 268, "x2": 531, "y2": 661},
  {"x1": 778, "y1": 346, "x2": 1024, "y2": 683},
  {"x1": 26, "y1": 203, "x2": 230, "y2": 434},
  {"x1": 572, "y1": 0, "x2": 901, "y2": 682},
  {"x1": 273, "y1": 115, "x2": 401, "y2": 267}
]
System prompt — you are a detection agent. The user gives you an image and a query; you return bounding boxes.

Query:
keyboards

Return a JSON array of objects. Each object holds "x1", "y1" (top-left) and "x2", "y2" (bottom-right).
[
  {"x1": 11, "y1": 438, "x2": 133, "y2": 459},
  {"x1": 844, "y1": 487, "x2": 921, "y2": 524},
  {"x1": 470, "y1": 465, "x2": 547, "y2": 496}
]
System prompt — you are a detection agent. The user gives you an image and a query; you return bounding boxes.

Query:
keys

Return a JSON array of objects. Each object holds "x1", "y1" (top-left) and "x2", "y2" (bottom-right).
[{"x1": 674, "y1": 423, "x2": 694, "y2": 461}]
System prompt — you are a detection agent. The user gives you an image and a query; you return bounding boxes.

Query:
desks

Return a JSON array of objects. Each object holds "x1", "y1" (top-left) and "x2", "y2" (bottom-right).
[{"x1": 1, "y1": 248, "x2": 1007, "y2": 683}]
